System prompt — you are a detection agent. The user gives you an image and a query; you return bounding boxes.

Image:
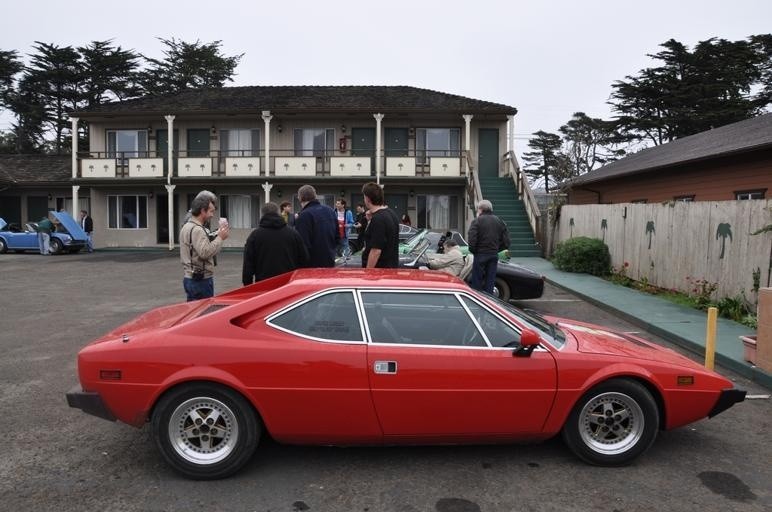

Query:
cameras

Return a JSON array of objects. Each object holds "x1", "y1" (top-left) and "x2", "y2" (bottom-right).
[{"x1": 193, "y1": 268, "x2": 204, "y2": 280}]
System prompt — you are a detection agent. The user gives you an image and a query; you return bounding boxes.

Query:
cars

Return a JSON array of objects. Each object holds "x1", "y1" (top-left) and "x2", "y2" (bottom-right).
[{"x1": 0, "y1": 211, "x2": 90, "y2": 255}]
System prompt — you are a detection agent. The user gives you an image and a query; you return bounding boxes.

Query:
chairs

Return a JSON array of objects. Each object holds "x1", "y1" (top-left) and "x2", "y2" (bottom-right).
[
  {"x1": 459, "y1": 254, "x2": 474, "y2": 280},
  {"x1": 374, "y1": 301, "x2": 440, "y2": 344},
  {"x1": 13, "y1": 227, "x2": 21, "y2": 232}
]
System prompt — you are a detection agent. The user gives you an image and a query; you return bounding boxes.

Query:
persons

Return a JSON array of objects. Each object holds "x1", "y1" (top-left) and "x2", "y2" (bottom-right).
[
  {"x1": 178, "y1": 194, "x2": 233, "y2": 304},
  {"x1": 362, "y1": 181, "x2": 401, "y2": 269},
  {"x1": 53, "y1": 208, "x2": 67, "y2": 232},
  {"x1": 37, "y1": 215, "x2": 57, "y2": 256},
  {"x1": 424, "y1": 235, "x2": 466, "y2": 279},
  {"x1": 241, "y1": 201, "x2": 310, "y2": 287},
  {"x1": 279, "y1": 198, "x2": 373, "y2": 259},
  {"x1": 295, "y1": 184, "x2": 342, "y2": 269},
  {"x1": 467, "y1": 199, "x2": 510, "y2": 296},
  {"x1": 80, "y1": 209, "x2": 93, "y2": 252},
  {"x1": 181, "y1": 190, "x2": 231, "y2": 267}
]
